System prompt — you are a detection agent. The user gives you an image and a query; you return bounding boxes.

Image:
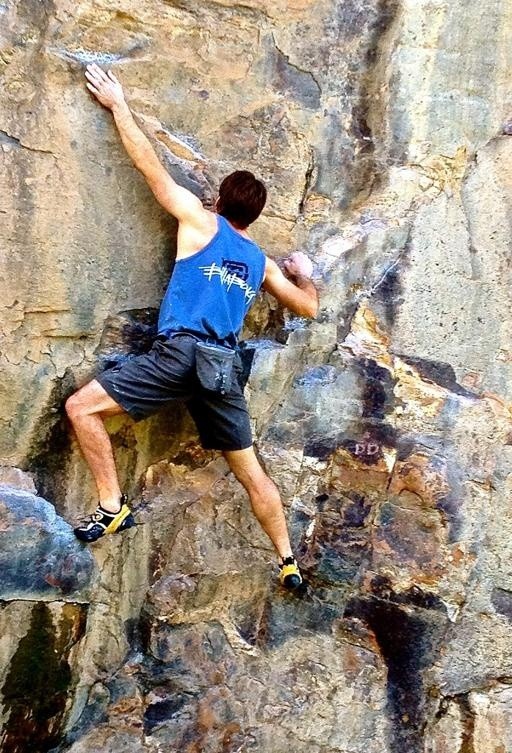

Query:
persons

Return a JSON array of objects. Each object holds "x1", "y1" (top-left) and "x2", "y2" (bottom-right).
[{"x1": 62, "y1": 62, "x2": 321, "y2": 589}]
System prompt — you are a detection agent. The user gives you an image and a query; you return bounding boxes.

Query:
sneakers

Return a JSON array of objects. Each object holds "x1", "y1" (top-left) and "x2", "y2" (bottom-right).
[
  {"x1": 276, "y1": 554, "x2": 304, "y2": 593},
  {"x1": 74, "y1": 492, "x2": 134, "y2": 542}
]
